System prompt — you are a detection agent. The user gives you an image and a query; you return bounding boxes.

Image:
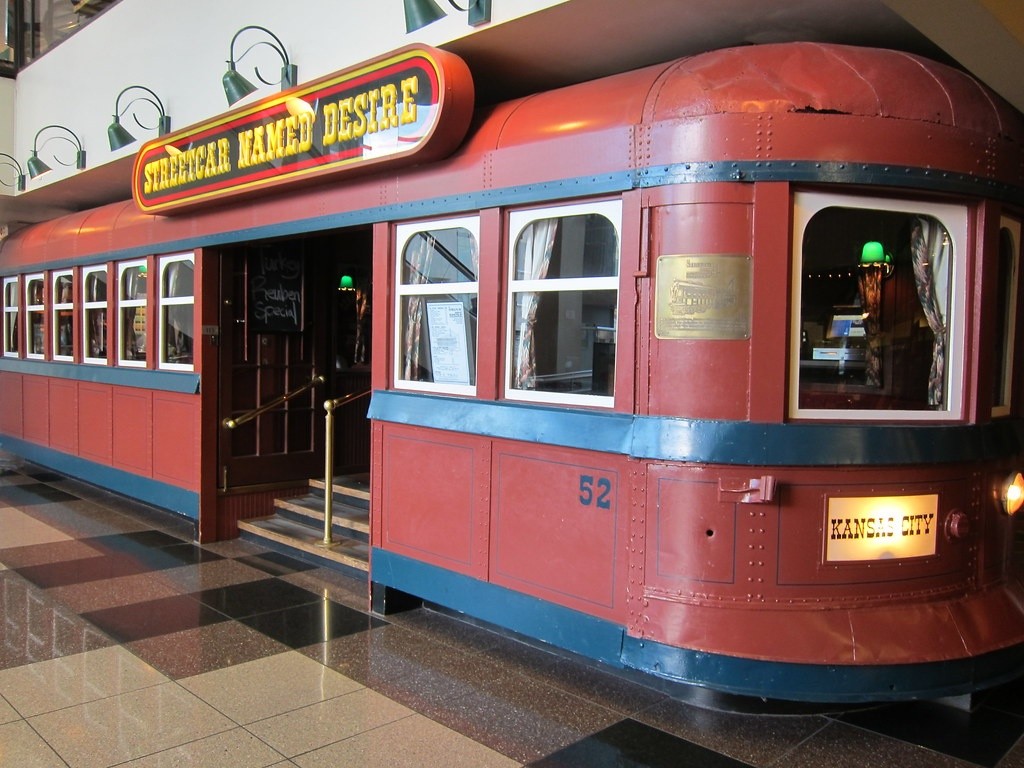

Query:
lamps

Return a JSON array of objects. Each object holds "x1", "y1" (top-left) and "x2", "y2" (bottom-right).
[
  {"x1": 27, "y1": 125, "x2": 86, "y2": 179},
  {"x1": 337, "y1": 275, "x2": 356, "y2": 291},
  {"x1": 222, "y1": 25, "x2": 298, "y2": 107},
  {"x1": 0, "y1": 152, "x2": 26, "y2": 191},
  {"x1": 107, "y1": 86, "x2": 171, "y2": 152},
  {"x1": 403, "y1": 0, "x2": 493, "y2": 34},
  {"x1": 858, "y1": 241, "x2": 896, "y2": 278}
]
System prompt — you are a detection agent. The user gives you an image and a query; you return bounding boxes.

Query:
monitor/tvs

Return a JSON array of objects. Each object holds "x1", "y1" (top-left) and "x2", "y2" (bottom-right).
[{"x1": 826, "y1": 305, "x2": 866, "y2": 340}]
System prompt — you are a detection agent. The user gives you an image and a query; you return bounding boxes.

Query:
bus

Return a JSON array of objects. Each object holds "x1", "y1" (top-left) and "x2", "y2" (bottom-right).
[{"x1": 0, "y1": 41, "x2": 1024, "y2": 714}]
